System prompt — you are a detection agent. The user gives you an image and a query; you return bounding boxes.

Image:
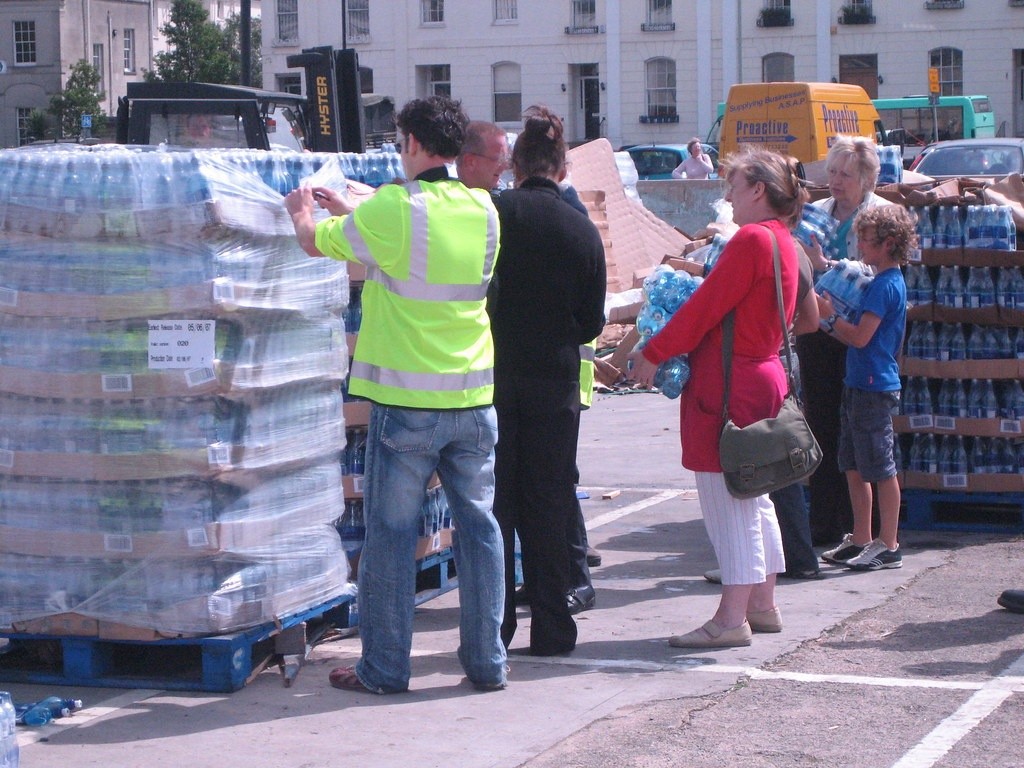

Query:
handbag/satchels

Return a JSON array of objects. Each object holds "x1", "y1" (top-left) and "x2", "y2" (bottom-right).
[{"x1": 718, "y1": 395, "x2": 823, "y2": 497}]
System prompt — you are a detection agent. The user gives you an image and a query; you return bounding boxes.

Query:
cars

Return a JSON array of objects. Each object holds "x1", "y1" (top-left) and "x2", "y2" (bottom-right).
[
  {"x1": 908, "y1": 137, "x2": 1024, "y2": 182},
  {"x1": 623, "y1": 143, "x2": 719, "y2": 180}
]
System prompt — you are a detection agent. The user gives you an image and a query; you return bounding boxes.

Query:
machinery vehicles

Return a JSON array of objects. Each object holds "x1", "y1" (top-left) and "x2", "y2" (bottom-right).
[{"x1": 114, "y1": 45, "x2": 365, "y2": 155}]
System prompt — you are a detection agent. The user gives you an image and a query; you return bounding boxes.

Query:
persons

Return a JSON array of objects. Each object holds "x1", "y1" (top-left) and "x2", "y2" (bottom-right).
[
  {"x1": 811, "y1": 135, "x2": 893, "y2": 544},
  {"x1": 457, "y1": 119, "x2": 511, "y2": 192},
  {"x1": 286, "y1": 97, "x2": 508, "y2": 694},
  {"x1": 187, "y1": 113, "x2": 210, "y2": 147},
  {"x1": 814, "y1": 204, "x2": 917, "y2": 570},
  {"x1": 627, "y1": 149, "x2": 820, "y2": 648},
  {"x1": 672, "y1": 138, "x2": 714, "y2": 180},
  {"x1": 491, "y1": 107, "x2": 607, "y2": 655}
]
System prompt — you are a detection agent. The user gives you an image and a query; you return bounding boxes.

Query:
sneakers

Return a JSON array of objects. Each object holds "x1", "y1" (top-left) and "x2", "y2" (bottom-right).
[
  {"x1": 821, "y1": 533, "x2": 872, "y2": 564},
  {"x1": 474, "y1": 676, "x2": 503, "y2": 690},
  {"x1": 847, "y1": 538, "x2": 903, "y2": 569},
  {"x1": 329, "y1": 665, "x2": 373, "y2": 691}
]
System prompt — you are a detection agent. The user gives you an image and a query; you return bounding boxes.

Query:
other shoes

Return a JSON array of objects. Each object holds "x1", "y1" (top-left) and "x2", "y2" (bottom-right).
[
  {"x1": 703, "y1": 569, "x2": 721, "y2": 584},
  {"x1": 587, "y1": 546, "x2": 601, "y2": 566},
  {"x1": 778, "y1": 566, "x2": 817, "y2": 579},
  {"x1": 998, "y1": 590, "x2": 1024, "y2": 613},
  {"x1": 746, "y1": 606, "x2": 782, "y2": 632},
  {"x1": 669, "y1": 620, "x2": 752, "y2": 647},
  {"x1": 566, "y1": 585, "x2": 596, "y2": 615}
]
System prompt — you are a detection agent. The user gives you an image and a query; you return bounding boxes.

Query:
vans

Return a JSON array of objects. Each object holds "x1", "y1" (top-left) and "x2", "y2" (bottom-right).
[{"x1": 717, "y1": 81, "x2": 905, "y2": 178}]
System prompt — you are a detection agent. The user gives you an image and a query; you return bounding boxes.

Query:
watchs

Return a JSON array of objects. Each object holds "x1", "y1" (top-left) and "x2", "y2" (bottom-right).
[
  {"x1": 827, "y1": 314, "x2": 839, "y2": 326},
  {"x1": 825, "y1": 260, "x2": 832, "y2": 272}
]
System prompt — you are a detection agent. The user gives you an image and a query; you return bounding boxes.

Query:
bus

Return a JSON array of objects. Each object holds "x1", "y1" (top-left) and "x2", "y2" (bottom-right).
[{"x1": 706, "y1": 95, "x2": 996, "y2": 174}]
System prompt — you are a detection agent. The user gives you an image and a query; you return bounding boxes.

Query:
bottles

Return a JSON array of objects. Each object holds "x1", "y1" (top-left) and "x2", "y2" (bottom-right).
[
  {"x1": 875, "y1": 145, "x2": 904, "y2": 185},
  {"x1": 0, "y1": 141, "x2": 454, "y2": 626},
  {"x1": 628, "y1": 232, "x2": 730, "y2": 401},
  {"x1": 892, "y1": 204, "x2": 1024, "y2": 475},
  {"x1": 790, "y1": 203, "x2": 875, "y2": 330},
  {"x1": 0, "y1": 691, "x2": 82, "y2": 768}
]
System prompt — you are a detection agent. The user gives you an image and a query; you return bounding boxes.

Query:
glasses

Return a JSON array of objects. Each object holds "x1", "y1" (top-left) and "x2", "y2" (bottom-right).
[{"x1": 474, "y1": 153, "x2": 511, "y2": 163}]
